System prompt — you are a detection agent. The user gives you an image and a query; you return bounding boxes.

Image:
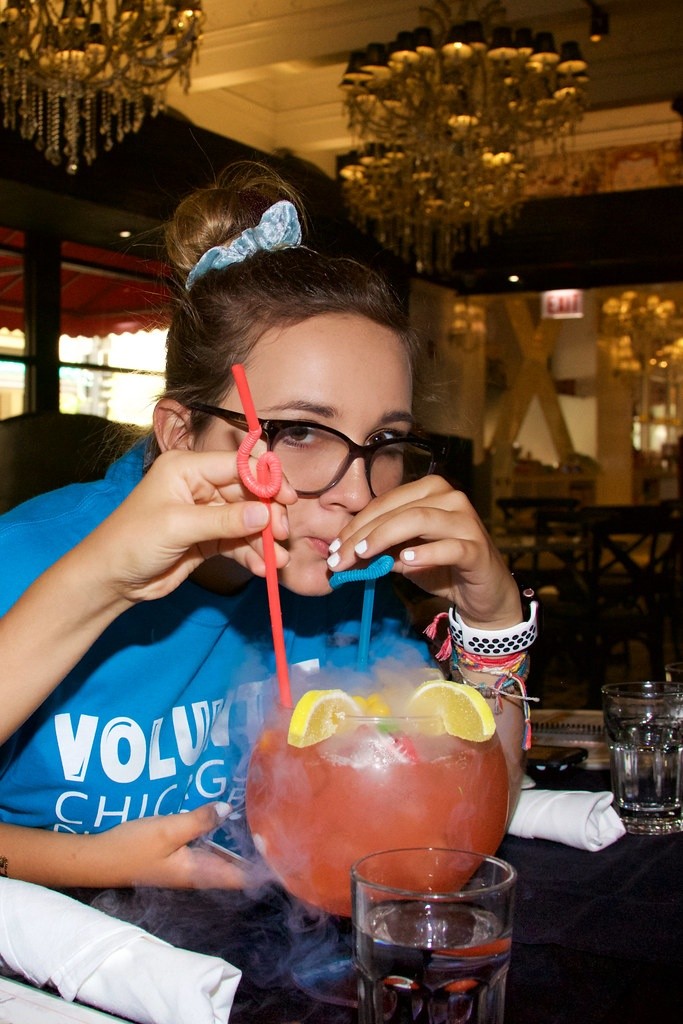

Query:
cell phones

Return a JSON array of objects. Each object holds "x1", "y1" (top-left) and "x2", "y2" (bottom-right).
[
  {"x1": 523, "y1": 745, "x2": 589, "y2": 772},
  {"x1": 201, "y1": 807, "x2": 274, "y2": 877}
]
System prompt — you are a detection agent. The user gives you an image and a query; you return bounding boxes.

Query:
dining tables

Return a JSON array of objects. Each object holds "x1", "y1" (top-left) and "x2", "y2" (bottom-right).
[{"x1": 83, "y1": 703, "x2": 683, "y2": 1023}]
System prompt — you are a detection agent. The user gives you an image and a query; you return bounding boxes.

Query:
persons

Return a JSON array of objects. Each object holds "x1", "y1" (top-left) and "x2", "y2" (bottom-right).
[
  {"x1": 0, "y1": 171, "x2": 538, "y2": 890},
  {"x1": 0, "y1": 449, "x2": 298, "y2": 749}
]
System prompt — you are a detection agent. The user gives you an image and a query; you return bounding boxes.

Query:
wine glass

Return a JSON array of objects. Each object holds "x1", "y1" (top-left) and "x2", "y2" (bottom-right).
[{"x1": 245, "y1": 676, "x2": 509, "y2": 1007}]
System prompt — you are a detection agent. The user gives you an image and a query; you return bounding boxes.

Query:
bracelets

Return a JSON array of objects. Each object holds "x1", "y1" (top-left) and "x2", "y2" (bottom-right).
[{"x1": 423, "y1": 612, "x2": 540, "y2": 751}]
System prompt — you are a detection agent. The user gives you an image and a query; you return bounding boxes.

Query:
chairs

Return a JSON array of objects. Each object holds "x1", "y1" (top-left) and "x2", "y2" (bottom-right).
[{"x1": 496, "y1": 496, "x2": 683, "y2": 708}]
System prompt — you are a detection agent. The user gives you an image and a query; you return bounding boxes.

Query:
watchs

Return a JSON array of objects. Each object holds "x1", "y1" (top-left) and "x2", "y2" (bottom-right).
[{"x1": 447, "y1": 564, "x2": 537, "y2": 655}]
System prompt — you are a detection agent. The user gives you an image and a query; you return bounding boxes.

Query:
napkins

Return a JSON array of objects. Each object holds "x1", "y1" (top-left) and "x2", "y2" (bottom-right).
[
  {"x1": 508, "y1": 789, "x2": 627, "y2": 852},
  {"x1": 0, "y1": 875, "x2": 242, "y2": 1024}
]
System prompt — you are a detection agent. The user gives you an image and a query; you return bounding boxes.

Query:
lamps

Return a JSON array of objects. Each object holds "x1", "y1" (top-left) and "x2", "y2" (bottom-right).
[
  {"x1": 590, "y1": 7, "x2": 608, "y2": 42},
  {"x1": 0, "y1": 0, "x2": 206, "y2": 177},
  {"x1": 335, "y1": 1, "x2": 592, "y2": 277}
]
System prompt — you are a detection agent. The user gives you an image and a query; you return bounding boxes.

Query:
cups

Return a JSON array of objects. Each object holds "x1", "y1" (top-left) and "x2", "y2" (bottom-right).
[
  {"x1": 601, "y1": 682, "x2": 683, "y2": 835},
  {"x1": 350, "y1": 847, "x2": 518, "y2": 1024}
]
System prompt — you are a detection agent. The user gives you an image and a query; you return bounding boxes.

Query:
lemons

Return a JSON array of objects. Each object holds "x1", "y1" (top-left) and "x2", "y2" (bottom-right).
[
  {"x1": 287, "y1": 688, "x2": 366, "y2": 748},
  {"x1": 404, "y1": 681, "x2": 496, "y2": 742}
]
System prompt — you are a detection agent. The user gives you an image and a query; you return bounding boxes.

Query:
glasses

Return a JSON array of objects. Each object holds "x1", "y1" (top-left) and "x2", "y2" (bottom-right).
[{"x1": 187, "y1": 401, "x2": 448, "y2": 500}]
